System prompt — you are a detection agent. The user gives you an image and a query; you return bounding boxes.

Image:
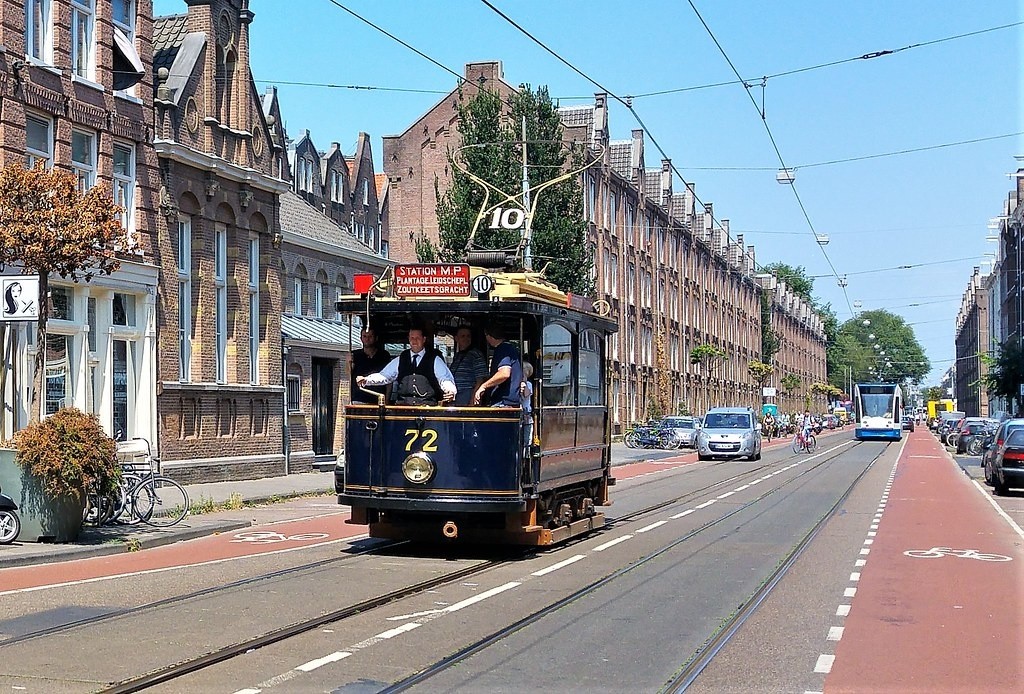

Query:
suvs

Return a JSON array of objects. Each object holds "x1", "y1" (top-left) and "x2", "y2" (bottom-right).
[
  {"x1": 981, "y1": 419, "x2": 1024, "y2": 485},
  {"x1": 699, "y1": 405, "x2": 765, "y2": 459}
]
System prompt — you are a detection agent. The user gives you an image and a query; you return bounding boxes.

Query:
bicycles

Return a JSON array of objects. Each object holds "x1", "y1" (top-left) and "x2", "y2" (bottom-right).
[
  {"x1": 792, "y1": 427, "x2": 821, "y2": 454},
  {"x1": 622, "y1": 421, "x2": 685, "y2": 449},
  {"x1": 766, "y1": 426, "x2": 772, "y2": 442},
  {"x1": 80, "y1": 432, "x2": 188, "y2": 529}
]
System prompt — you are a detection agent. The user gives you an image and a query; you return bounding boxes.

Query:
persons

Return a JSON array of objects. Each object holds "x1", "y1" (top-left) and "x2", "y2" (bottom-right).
[
  {"x1": 356, "y1": 319, "x2": 457, "y2": 403},
  {"x1": 763, "y1": 412, "x2": 775, "y2": 434},
  {"x1": 473, "y1": 319, "x2": 523, "y2": 409},
  {"x1": 520, "y1": 362, "x2": 534, "y2": 459},
  {"x1": 914, "y1": 414, "x2": 920, "y2": 423},
  {"x1": 780, "y1": 411, "x2": 820, "y2": 433},
  {"x1": 450, "y1": 325, "x2": 490, "y2": 406},
  {"x1": 346, "y1": 323, "x2": 394, "y2": 406},
  {"x1": 802, "y1": 409, "x2": 815, "y2": 444}
]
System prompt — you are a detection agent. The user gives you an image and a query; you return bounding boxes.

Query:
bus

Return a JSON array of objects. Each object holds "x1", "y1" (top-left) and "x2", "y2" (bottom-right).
[
  {"x1": 854, "y1": 383, "x2": 906, "y2": 439},
  {"x1": 924, "y1": 400, "x2": 955, "y2": 427},
  {"x1": 337, "y1": 139, "x2": 619, "y2": 547}
]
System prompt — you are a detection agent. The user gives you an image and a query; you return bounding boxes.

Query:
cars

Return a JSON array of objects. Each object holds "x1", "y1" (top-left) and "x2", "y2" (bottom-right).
[
  {"x1": 901, "y1": 416, "x2": 917, "y2": 432},
  {"x1": 994, "y1": 429, "x2": 1024, "y2": 496},
  {"x1": 658, "y1": 416, "x2": 700, "y2": 449},
  {"x1": 761, "y1": 414, "x2": 844, "y2": 434},
  {"x1": 934, "y1": 411, "x2": 1013, "y2": 456}
]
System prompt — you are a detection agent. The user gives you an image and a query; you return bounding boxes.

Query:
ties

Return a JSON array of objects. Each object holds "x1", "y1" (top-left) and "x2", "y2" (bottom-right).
[{"x1": 412, "y1": 354, "x2": 419, "y2": 368}]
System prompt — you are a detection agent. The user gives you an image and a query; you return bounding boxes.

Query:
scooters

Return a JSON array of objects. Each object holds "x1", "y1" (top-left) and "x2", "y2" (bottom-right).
[{"x1": 0, "y1": 486, "x2": 22, "y2": 544}]
